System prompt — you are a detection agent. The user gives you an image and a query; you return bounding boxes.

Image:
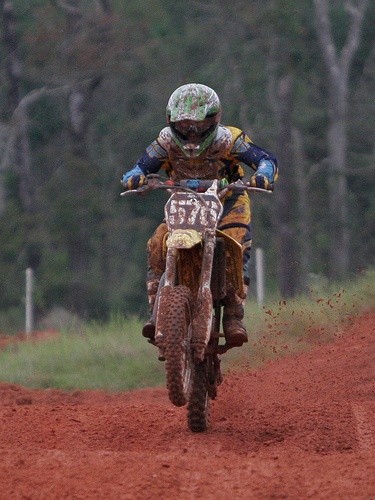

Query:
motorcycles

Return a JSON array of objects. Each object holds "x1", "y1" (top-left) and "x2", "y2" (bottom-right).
[{"x1": 119, "y1": 173, "x2": 274, "y2": 434}]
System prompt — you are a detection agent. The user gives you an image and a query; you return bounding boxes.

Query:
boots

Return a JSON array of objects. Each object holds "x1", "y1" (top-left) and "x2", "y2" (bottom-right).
[
  {"x1": 142, "y1": 279, "x2": 160, "y2": 341},
  {"x1": 222, "y1": 276, "x2": 250, "y2": 343}
]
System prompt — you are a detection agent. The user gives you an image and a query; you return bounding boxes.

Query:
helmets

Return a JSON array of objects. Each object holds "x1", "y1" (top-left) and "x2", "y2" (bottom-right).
[{"x1": 166, "y1": 83, "x2": 221, "y2": 158}]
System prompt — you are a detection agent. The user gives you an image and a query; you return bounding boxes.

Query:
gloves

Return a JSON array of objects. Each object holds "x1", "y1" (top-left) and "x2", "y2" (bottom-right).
[
  {"x1": 250, "y1": 174, "x2": 272, "y2": 194},
  {"x1": 121, "y1": 172, "x2": 146, "y2": 195}
]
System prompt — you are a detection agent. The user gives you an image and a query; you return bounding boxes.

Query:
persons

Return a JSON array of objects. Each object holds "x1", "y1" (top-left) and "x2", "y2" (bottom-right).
[{"x1": 119, "y1": 82, "x2": 280, "y2": 351}]
